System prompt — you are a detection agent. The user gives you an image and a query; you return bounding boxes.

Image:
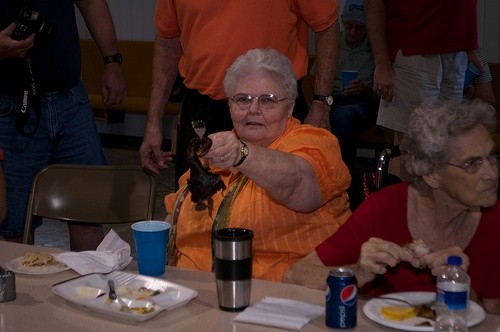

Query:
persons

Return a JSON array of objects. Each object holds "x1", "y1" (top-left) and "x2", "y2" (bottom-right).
[
  {"x1": 363, "y1": 0, "x2": 483, "y2": 190},
  {"x1": 164, "y1": 48, "x2": 352, "y2": 284},
  {"x1": 309, "y1": 0, "x2": 401, "y2": 189},
  {"x1": 139, "y1": 0, "x2": 342, "y2": 193},
  {"x1": 0, "y1": 0, "x2": 126, "y2": 253},
  {"x1": 463, "y1": 46, "x2": 496, "y2": 106},
  {"x1": 281, "y1": 96, "x2": 500, "y2": 318}
]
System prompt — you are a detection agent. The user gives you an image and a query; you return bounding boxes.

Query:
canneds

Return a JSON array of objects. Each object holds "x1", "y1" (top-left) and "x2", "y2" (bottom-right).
[{"x1": 325, "y1": 268, "x2": 359, "y2": 330}]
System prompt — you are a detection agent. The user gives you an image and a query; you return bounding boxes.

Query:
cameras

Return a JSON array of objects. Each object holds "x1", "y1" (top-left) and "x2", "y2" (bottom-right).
[{"x1": 11, "y1": 9, "x2": 51, "y2": 41}]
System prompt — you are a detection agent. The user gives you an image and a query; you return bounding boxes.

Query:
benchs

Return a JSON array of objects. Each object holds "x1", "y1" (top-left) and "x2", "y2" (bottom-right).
[{"x1": 78, "y1": 39, "x2": 181, "y2": 157}]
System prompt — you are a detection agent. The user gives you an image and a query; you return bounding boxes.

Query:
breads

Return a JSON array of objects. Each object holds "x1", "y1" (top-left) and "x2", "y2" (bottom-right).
[
  {"x1": 23, "y1": 250, "x2": 56, "y2": 267},
  {"x1": 408, "y1": 239, "x2": 431, "y2": 259}
]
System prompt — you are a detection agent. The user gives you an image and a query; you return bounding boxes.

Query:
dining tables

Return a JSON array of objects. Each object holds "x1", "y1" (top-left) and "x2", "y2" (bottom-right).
[{"x1": 0, "y1": 240, "x2": 500, "y2": 332}]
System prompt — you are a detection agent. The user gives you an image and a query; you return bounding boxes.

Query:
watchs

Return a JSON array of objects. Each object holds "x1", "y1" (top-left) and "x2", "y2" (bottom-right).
[
  {"x1": 103, "y1": 53, "x2": 123, "y2": 64},
  {"x1": 312, "y1": 95, "x2": 334, "y2": 106},
  {"x1": 233, "y1": 141, "x2": 249, "y2": 167}
]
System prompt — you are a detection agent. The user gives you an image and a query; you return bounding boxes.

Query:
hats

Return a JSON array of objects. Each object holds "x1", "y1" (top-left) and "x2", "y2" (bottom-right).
[{"x1": 343, "y1": 0, "x2": 367, "y2": 24}]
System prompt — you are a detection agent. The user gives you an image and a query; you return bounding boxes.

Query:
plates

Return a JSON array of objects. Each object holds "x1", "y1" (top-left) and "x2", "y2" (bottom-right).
[
  {"x1": 6, "y1": 253, "x2": 72, "y2": 275},
  {"x1": 362, "y1": 291, "x2": 486, "y2": 332},
  {"x1": 51, "y1": 270, "x2": 199, "y2": 322}
]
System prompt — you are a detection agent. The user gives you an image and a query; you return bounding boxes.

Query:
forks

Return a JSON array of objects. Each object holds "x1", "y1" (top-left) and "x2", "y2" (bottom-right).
[{"x1": 360, "y1": 296, "x2": 436, "y2": 308}]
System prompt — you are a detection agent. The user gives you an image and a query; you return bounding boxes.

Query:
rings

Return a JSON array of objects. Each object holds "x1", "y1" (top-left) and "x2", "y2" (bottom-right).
[
  {"x1": 221, "y1": 156, "x2": 225, "y2": 161},
  {"x1": 142, "y1": 168, "x2": 148, "y2": 174},
  {"x1": 378, "y1": 89, "x2": 382, "y2": 92}
]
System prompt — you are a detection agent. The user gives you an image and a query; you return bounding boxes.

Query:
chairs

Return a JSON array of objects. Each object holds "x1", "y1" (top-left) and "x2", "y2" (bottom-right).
[{"x1": 20, "y1": 163, "x2": 155, "y2": 246}]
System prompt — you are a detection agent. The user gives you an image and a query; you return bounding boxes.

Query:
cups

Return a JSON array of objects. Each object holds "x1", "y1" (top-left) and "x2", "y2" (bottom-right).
[
  {"x1": 212, "y1": 228, "x2": 254, "y2": 313},
  {"x1": 131, "y1": 220, "x2": 171, "y2": 277}
]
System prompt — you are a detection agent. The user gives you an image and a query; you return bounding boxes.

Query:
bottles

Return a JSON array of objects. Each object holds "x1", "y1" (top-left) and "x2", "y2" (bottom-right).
[{"x1": 435, "y1": 255, "x2": 471, "y2": 332}]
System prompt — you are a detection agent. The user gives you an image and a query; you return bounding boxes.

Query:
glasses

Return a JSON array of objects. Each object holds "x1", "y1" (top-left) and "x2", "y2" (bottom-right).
[
  {"x1": 226, "y1": 93, "x2": 290, "y2": 112},
  {"x1": 439, "y1": 152, "x2": 498, "y2": 174}
]
